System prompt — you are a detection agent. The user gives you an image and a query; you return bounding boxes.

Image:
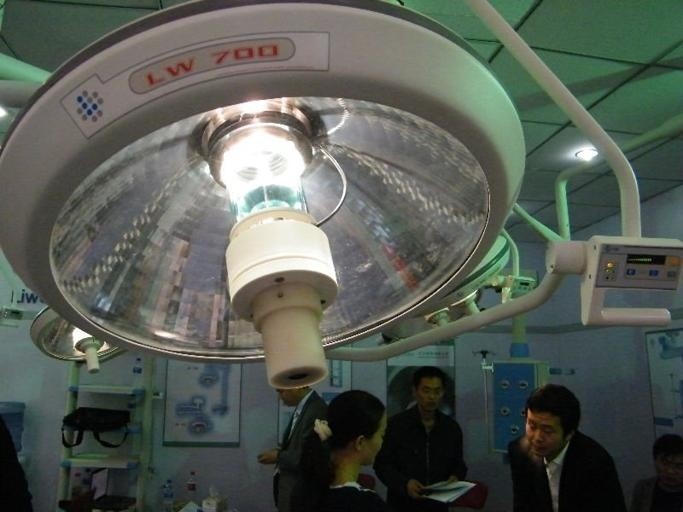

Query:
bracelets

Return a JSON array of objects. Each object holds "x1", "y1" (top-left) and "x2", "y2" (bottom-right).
[{"x1": 276, "y1": 450, "x2": 281, "y2": 461}]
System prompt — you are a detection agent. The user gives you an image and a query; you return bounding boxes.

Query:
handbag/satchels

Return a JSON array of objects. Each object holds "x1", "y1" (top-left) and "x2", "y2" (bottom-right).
[{"x1": 60, "y1": 406, "x2": 132, "y2": 448}]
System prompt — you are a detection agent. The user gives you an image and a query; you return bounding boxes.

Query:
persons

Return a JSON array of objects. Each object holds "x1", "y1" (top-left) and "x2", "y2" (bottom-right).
[
  {"x1": 286, "y1": 388, "x2": 392, "y2": 512},
  {"x1": 630, "y1": 432, "x2": 682, "y2": 511},
  {"x1": 372, "y1": 364, "x2": 470, "y2": 511},
  {"x1": 253, "y1": 385, "x2": 328, "y2": 511},
  {"x1": 504, "y1": 381, "x2": 625, "y2": 511}
]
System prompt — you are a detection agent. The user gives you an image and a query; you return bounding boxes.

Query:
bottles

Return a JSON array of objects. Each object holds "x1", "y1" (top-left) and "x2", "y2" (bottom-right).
[
  {"x1": 132, "y1": 357, "x2": 144, "y2": 389},
  {"x1": 161, "y1": 480, "x2": 176, "y2": 512},
  {"x1": 186, "y1": 471, "x2": 198, "y2": 505},
  {"x1": 80, "y1": 468, "x2": 92, "y2": 494},
  {"x1": 69, "y1": 472, "x2": 82, "y2": 499}
]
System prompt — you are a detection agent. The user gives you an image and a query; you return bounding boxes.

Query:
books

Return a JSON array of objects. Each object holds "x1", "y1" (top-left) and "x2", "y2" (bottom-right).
[{"x1": 416, "y1": 479, "x2": 477, "y2": 503}]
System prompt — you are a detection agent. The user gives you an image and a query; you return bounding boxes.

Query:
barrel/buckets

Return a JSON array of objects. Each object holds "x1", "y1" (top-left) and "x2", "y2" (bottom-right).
[{"x1": 0, "y1": 402, "x2": 25, "y2": 452}]
